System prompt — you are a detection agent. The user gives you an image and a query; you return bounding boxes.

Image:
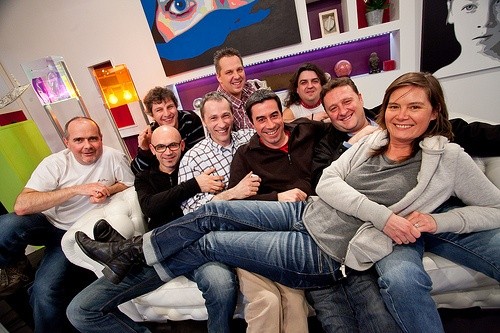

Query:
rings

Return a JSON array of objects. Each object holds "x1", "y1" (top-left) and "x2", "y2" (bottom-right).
[{"x1": 414, "y1": 224, "x2": 419, "y2": 228}]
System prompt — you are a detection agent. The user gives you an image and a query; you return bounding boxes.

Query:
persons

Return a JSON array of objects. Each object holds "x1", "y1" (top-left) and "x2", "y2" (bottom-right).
[
  {"x1": 74, "y1": 72, "x2": 500, "y2": 288},
  {"x1": 0, "y1": 118, "x2": 134, "y2": 333},
  {"x1": 129, "y1": 48, "x2": 500, "y2": 333}
]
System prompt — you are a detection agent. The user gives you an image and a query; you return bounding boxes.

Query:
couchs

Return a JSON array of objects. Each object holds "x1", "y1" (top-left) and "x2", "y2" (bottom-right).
[{"x1": 61, "y1": 157, "x2": 500, "y2": 323}]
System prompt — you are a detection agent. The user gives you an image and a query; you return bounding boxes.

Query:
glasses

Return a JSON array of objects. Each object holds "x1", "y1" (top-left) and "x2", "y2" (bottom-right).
[{"x1": 150, "y1": 141, "x2": 183, "y2": 152}]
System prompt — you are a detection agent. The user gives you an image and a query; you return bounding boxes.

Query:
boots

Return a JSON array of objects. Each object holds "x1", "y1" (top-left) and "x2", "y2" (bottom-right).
[
  {"x1": 93, "y1": 219, "x2": 126, "y2": 243},
  {"x1": 75, "y1": 231, "x2": 147, "y2": 284}
]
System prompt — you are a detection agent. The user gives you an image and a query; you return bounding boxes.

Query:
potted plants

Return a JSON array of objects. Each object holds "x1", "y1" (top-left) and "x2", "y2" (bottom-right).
[{"x1": 365, "y1": 0, "x2": 391, "y2": 26}]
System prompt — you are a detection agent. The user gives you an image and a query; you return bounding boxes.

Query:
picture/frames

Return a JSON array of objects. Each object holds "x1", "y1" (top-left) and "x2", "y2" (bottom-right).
[{"x1": 318, "y1": 6, "x2": 341, "y2": 39}]
